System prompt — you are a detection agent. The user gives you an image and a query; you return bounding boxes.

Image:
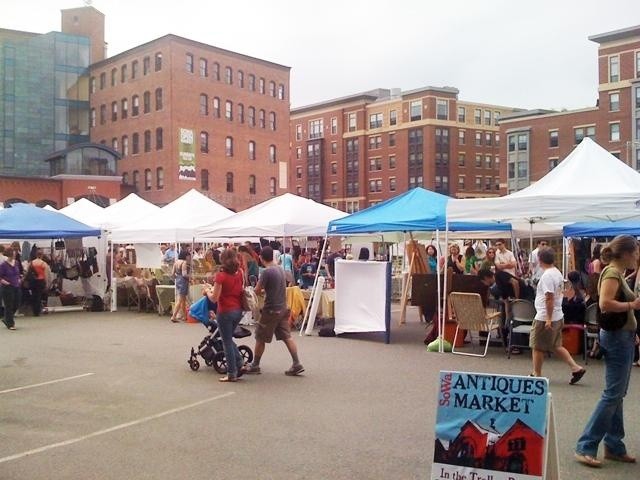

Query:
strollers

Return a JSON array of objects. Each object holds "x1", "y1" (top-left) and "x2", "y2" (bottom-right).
[{"x1": 185, "y1": 294, "x2": 254, "y2": 376}]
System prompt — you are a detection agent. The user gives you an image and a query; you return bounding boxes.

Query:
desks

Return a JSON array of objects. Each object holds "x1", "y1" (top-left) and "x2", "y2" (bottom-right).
[
  {"x1": 302, "y1": 289, "x2": 335, "y2": 326},
  {"x1": 156, "y1": 284, "x2": 203, "y2": 315}
]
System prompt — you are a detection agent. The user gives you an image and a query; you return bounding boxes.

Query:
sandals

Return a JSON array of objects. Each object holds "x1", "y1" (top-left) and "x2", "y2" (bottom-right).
[
  {"x1": 568, "y1": 368, "x2": 587, "y2": 384},
  {"x1": 573, "y1": 450, "x2": 604, "y2": 468},
  {"x1": 602, "y1": 447, "x2": 635, "y2": 462}
]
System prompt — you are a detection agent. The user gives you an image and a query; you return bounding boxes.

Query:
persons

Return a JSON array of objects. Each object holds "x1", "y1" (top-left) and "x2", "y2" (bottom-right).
[
  {"x1": 573, "y1": 236, "x2": 639, "y2": 467},
  {"x1": 106, "y1": 244, "x2": 370, "y2": 382},
  {"x1": 0, "y1": 243, "x2": 52, "y2": 330},
  {"x1": 425, "y1": 234, "x2": 639, "y2": 384}
]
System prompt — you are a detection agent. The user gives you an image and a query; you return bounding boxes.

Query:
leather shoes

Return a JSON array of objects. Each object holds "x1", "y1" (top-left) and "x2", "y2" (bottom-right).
[{"x1": 218, "y1": 376, "x2": 243, "y2": 382}]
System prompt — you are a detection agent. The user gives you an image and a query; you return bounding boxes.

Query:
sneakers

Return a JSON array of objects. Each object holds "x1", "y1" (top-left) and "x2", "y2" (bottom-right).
[
  {"x1": 242, "y1": 363, "x2": 261, "y2": 374},
  {"x1": 285, "y1": 364, "x2": 305, "y2": 376}
]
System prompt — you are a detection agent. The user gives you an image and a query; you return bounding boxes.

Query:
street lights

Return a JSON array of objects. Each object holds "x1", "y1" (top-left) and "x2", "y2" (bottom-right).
[{"x1": 626, "y1": 140, "x2": 639, "y2": 167}]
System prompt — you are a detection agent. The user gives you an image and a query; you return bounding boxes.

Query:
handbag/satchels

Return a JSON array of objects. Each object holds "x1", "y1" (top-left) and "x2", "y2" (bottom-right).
[
  {"x1": 55, "y1": 236, "x2": 65, "y2": 250},
  {"x1": 595, "y1": 285, "x2": 629, "y2": 332},
  {"x1": 26, "y1": 261, "x2": 39, "y2": 281},
  {"x1": 188, "y1": 294, "x2": 211, "y2": 328},
  {"x1": 42, "y1": 249, "x2": 99, "y2": 280},
  {"x1": 239, "y1": 286, "x2": 260, "y2": 311}
]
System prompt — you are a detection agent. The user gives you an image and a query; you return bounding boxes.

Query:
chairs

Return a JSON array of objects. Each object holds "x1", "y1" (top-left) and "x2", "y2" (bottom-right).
[
  {"x1": 509, "y1": 299, "x2": 552, "y2": 358},
  {"x1": 450, "y1": 292, "x2": 507, "y2": 358},
  {"x1": 584, "y1": 302, "x2": 598, "y2": 365}
]
